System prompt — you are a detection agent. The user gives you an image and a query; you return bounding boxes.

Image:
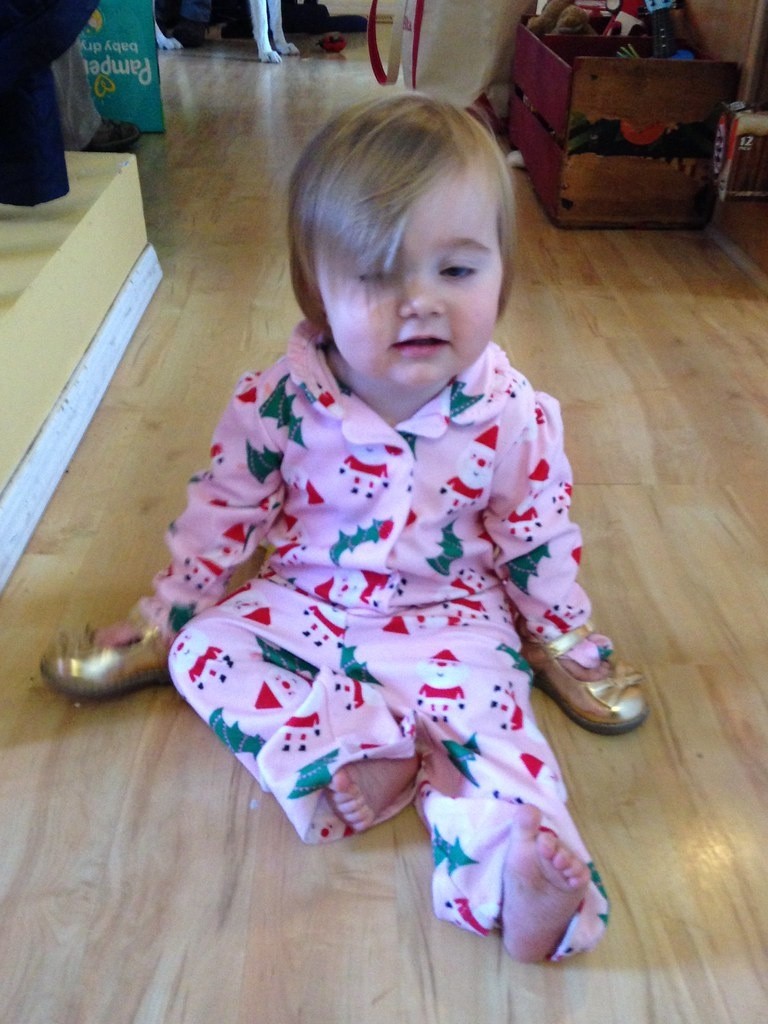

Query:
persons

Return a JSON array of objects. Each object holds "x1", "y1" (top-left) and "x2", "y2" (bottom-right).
[
  {"x1": 50, "y1": 35, "x2": 142, "y2": 152},
  {"x1": 42, "y1": 93, "x2": 646, "y2": 964}
]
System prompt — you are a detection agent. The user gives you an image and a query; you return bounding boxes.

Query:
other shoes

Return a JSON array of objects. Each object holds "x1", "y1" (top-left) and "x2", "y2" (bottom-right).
[
  {"x1": 44, "y1": 609, "x2": 169, "y2": 701},
  {"x1": 514, "y1": 617, "x2": 649, "y2": 732},
  {"x1": 85, "y1": 119, "x2": 140, "y2": 152}
]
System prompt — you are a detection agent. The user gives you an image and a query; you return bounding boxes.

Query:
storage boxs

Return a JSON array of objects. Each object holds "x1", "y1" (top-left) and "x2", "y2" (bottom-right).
[{"x1": 507, "y1": 13, "x2": 738, "y2": 233}]
